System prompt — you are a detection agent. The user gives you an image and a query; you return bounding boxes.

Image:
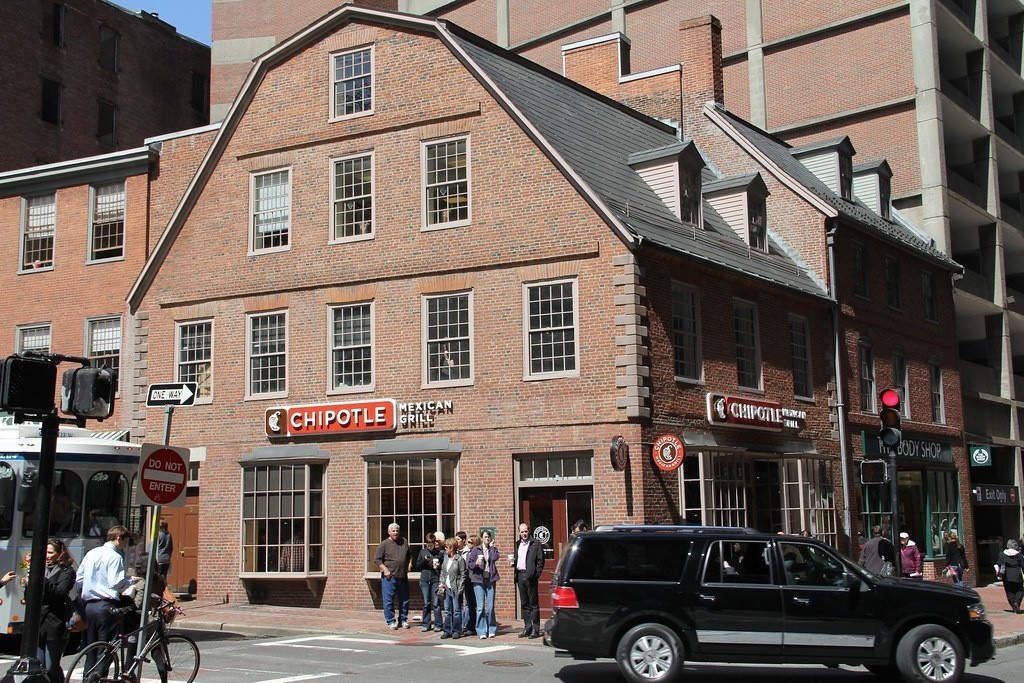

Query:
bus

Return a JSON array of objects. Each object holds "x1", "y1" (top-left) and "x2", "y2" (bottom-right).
[{"x1": 0, "y1": 420, "x2": 150, "y2": 646}]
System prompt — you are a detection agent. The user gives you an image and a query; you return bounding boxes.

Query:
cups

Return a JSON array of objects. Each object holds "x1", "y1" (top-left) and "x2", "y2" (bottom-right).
[
  {"x1": 508, "y1": 554, "x2": 514, "y2": 565},
  {"x1": 478, "y1": 555, "x2": 484, "y2": 564},
  {"x1": 433, "y1": 559, "x2": 439, "y2": 568}
]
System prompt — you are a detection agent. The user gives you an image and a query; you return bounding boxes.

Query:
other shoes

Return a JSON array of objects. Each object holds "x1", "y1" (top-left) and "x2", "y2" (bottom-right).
[
  {"x1": 441, "y1": 632, "x2": 452, "y2": 639},
  {"x1": 388, "y1": 622, "x2": 398, "y2": 630},
  {"x1": 422, "y1": 627, "x2": 431, "y2": 632},
  {"x1": 434, "y1": 626, "x2": 442, "y2": 632},
  {"x1": 529, "y1": 629, "x2": 539, "y2": 639},
  {"x1": 480, "y1": 635, "x2": 487, "y2": 639},
  {"x1": 518, "y1": 629, "x2": 532, "y2": 637},
  {"x1": 489, "y1": 633, "x2": 496, "y2": 638},
  {"x1": 398, "y1": 621, "x2": 410, "y2": 629},
  {"x1": 463, "y1": 627, "x2": 467, "y2": 632},
  {"x1": 464, "y1": 630, "x2": 477, "y2": 636},
  {"x1": 1014, "y1": 602, "x2": 1021, "y2": 614},
  {"x1": 453, "y1": 631, "x2": 461, "y2": 639}
]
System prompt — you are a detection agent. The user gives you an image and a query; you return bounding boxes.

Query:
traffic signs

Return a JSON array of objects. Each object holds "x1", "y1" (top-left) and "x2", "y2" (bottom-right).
[{"x1": 145, "y1": 381, "x2": 199, "y2": 408}]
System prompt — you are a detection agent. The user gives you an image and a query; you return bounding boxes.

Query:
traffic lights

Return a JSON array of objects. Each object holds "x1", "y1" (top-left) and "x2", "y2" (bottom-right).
[
  {"x1": 2, "y1": 357, "x2": 57, "y2": 414},
  {"x1": 878, "y1": 388, "x2": 903, "y2": 447},
  {"x1": 861, "y1": 460, "x2": 886, "y2": 484}
]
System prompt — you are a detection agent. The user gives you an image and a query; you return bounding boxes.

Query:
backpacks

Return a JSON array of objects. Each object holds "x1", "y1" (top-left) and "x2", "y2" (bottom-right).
[{"x1": 878, "y1": 540, "x2": 894, "y2": 561}]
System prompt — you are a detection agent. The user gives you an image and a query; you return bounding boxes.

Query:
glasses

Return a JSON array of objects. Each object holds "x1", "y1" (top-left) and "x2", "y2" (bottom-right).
[
  {"x1": 468, "y1": 541, "x2": 473, "y2": 544},
  {"x1": 445, "y1": 546, "x2": 448, "y2": 549}
]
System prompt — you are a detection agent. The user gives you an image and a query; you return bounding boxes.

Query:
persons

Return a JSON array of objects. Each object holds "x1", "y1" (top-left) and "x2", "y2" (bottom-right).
[
  {"x1": 438, "y1": 343, "x2": 455, "y2": 380},
  {"x1": 22, "y1": 540, "x2": 77, "y2": 683},
  {"x1": 0, "y1": 570, "x2": 18, "y2": 607},
  {"x1": 995, "y1": 533, "x2": 1024, "y2": 614},
  {"x1": 857, "y1": 526, "x2": 898, "y2": 578},
  {"x1": 418, "y1": 530, "x2": 498, "y2": 639},
  {"x1": 771, "y1": 530, "x2": 819, "y2": 579},
  {"x1": 895, "y1": 532, "x2": 922, "y2": 580},
  {"x1": 514, "y1": 523, "x2": 545, "y2": 639},
  {"x1": 723, "y1": 543, "x2": 749, "y2": 573},
  {"x1": 258, "y1": 527, "x2": 314, "y2": 572},
  {"x1": 142, "y1": 519, "x2": 173, "y2": 600},
  {"x1": 569, "y1": 519, "x2": 595, "y2": 535},
  {"x1": 374, "y1": 523, "x2": 412, "y2": 630},
  {"x1": 88, "y1": 518, "x2": 103, "y2": 538},
  {"x1": 74, "y1": 526, "x2": 141, "y2": 683},
  {"x1": 945, "y1": 532, "x2": 969, "y2": 589}
]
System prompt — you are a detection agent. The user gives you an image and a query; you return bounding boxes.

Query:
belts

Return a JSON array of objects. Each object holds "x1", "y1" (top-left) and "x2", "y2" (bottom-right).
[{"x1": 518, "y1": 570, "x2": 526, "y2": 573}]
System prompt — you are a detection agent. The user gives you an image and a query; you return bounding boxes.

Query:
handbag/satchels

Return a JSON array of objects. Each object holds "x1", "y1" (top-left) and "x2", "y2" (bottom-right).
[
  {"x1": 880, "y1": 561, "x2": 895, "y2": 577},
  {"x1": 161, "y1": 586, "x2": 180, "y2": 623},
  {"x1": 946, "y1": 567, "x2": 956, "y2": 578},
  {"x1": 65, "y1": 606, "x2": 89, "y2": 633},
  {"x1": 999, "y1": 564, "x2": 1005, "y2": 574}
]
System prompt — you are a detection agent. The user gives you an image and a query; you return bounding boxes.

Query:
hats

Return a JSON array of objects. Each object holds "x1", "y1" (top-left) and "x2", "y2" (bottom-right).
[{"x1": 900, "y1": 532, "x2": 909, "y2": 539}]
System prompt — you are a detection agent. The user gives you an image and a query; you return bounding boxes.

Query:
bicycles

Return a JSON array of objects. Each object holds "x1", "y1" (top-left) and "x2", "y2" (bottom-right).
[{"x1": 62, "y1": 593, "x2": 200, "y2": 683}]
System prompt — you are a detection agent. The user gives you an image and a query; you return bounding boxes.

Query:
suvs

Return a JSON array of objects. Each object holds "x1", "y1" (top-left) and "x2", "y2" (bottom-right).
[{"x1": 542, "y1": 524, "x2": 998, "y2": 683}]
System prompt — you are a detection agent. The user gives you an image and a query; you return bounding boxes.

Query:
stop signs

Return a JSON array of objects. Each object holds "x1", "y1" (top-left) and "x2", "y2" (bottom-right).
[{"x1": 134, "y1": 443, "x2": 191, "y2": 507}]
